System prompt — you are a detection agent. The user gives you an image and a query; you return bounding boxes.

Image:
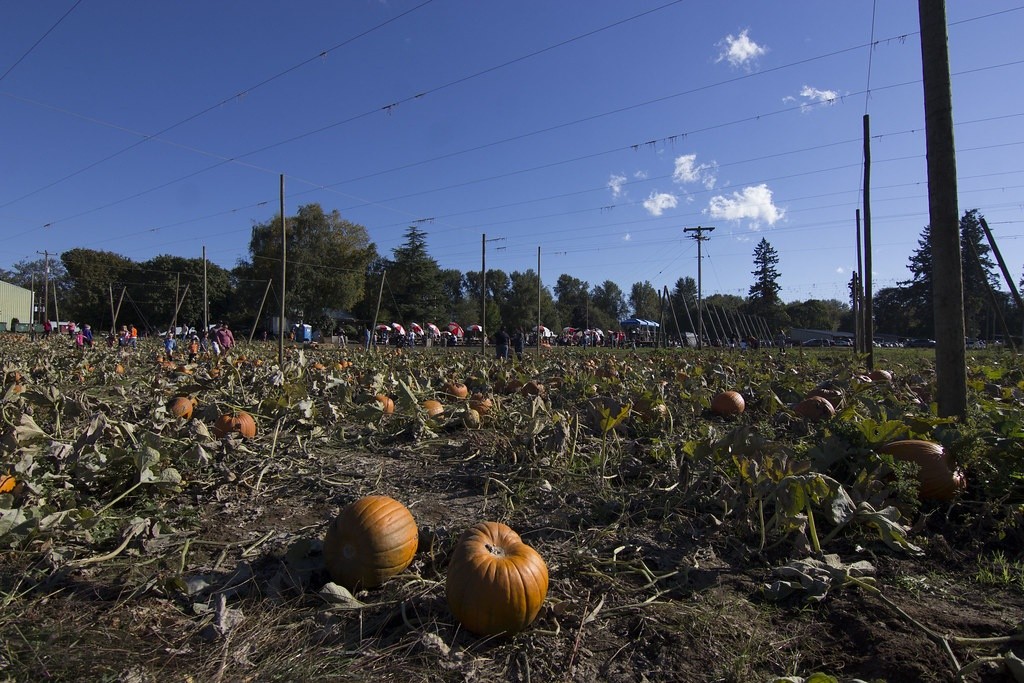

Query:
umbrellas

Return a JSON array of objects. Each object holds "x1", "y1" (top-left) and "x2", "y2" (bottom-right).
[
  {"x1": 467, "y1": 325, "x2": 479, "y2": 337},
  {"x1": 427, "y1": 323, "x2": 440, "y2": 336},
  {"x1": 562, "y1": 327, "x2": 575, "y2": 334},
  {"x1": 532, "y1": 325, "x2": 550, "y2": 333},
  {"x1": 375, "y1": 325, "x2": 391, "y2": 337},
  {"x1": 391, "y1": 323, "x2": 405, "y2": 336},
  {"x1": 409, "y1": 322, "x2": 424, "y2": 335},
  {"x1": 448, "y1": 322, "x2": 464, "y2": 337}
]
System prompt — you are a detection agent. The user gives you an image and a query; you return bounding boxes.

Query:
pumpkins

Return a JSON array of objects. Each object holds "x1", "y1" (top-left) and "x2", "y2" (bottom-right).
[
  {"x1": 0, "y1": 352, "x2": 495, "y2": 496},
  {"x1": 323, "y1": 496, "x2": 418, "y2": 588},
  {"x1": 445, "y1": 522, "x2": 549, "y2": 634},
  {"x1": 595, "y1": 368, "x2": 967, "y2": 505}
]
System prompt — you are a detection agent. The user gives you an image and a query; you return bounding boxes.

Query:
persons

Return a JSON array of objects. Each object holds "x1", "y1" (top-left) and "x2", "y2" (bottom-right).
[
  {"x1": 209, "y1": 319, "x2": 235, "y2": 356},
  {"x1": 777, "y1": 330, "x2": 786, "y2": 353},
  {"x1": 67, "y1": 320, "x2": 94, "y2": 351},
  {"x1": 364, "y1": 329, "x2": 371, "y2": 348},
  {"x1": 513, "y1": 325, "x2": 526, "y2": 360},
  {"x1": 289, "y1": 326, "x2": 296, "y2": 342},
  {"x1": 162, "y1": 333, "x2": 177, "y2": 360},
  {"x1": 581, "y1": 329, "x2": 627, "y2": 351},
  {"x1": 189, "y1": 332, "x2": 210, "y2": 361},
  {"x1": 729, "y1": 333, "x2": 736, "y2": 355},
  {"x1": 748, "y1": 335, "x2": 759, "y2": 349},
  {"x1": 373, "y1": 331, "x2": 378, "y2": 351},
  {"x1": 409, "y1": 330, "x2": 416, "y2": 346},
  {"x1": 105, "y1": 324, "x2": 137, "y2": 352},
  {"x1": 337, "y1": 327, "x2": 346, "y2": 347},
  {"x1": 41, "y1": 319, "x2": 52, "y2": 339},
  {"x1": 180, "y1": 324, "x2": 189, "y2": 340},
  {"x1": 490, "y1": 326, "x2": 511, "y2": 360},
  {"x1": 740, "y1": 339, "x2": 748, "y2": 351},
  {"x1": 448, "y1": 333, "x2": 457, "y2": 346}
]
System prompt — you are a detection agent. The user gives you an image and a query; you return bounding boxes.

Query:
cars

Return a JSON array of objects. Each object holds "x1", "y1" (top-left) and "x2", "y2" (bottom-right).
[
  {"x1": 800, "y1": 336, "x2": 854, "y2": 347},
  {"x1": 909, "y1": 338, "x2": 936, "y2": 348}
]
